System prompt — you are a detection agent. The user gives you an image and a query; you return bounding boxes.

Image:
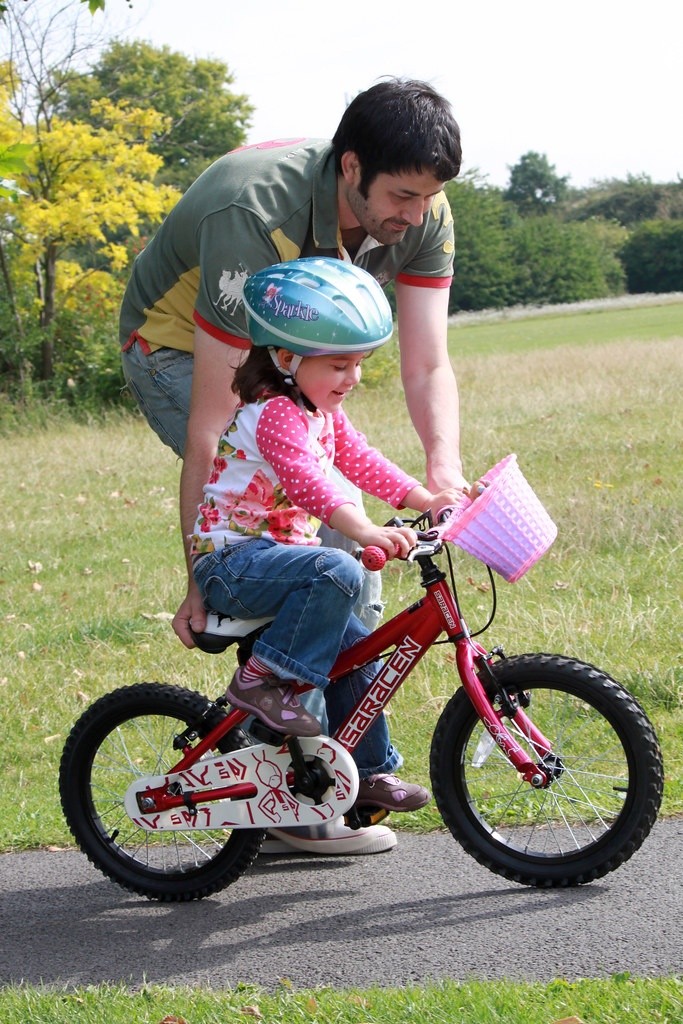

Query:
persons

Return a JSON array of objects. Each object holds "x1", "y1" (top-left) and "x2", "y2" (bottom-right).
[
  {"x1": 118, "y1": 81, "x2": 475, "y2": 855},
  {"x1": 190, "y1": 256, "x2": 472, "y2": 809}
]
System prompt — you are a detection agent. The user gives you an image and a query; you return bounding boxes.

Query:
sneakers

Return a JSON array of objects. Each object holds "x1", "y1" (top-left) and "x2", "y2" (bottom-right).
[
  {"x1": 355, "y1": 772, "x2": 432, "y2": 812},
  {"x1": 259, "y1": 815, "x2": 397, "y2": 853},
  {"x1": 225, "y1": 663, "x2": 321, "y2": 736}
]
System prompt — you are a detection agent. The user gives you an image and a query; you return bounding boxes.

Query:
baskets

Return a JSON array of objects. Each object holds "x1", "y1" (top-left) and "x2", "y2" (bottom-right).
[{"x1": 437, "y1": 455, "x2": 559, "y2": 582}]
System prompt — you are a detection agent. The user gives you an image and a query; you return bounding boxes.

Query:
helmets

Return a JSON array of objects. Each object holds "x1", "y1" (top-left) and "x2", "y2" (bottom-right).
[{"x1": 241, "y1": 256, "x2": 393, "y2": 357}]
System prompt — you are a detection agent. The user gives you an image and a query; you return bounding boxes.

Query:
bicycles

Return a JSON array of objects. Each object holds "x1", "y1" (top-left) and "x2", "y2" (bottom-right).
[{"x1": 60, "y1": 454, "x2": 665, "y2": 902}]
